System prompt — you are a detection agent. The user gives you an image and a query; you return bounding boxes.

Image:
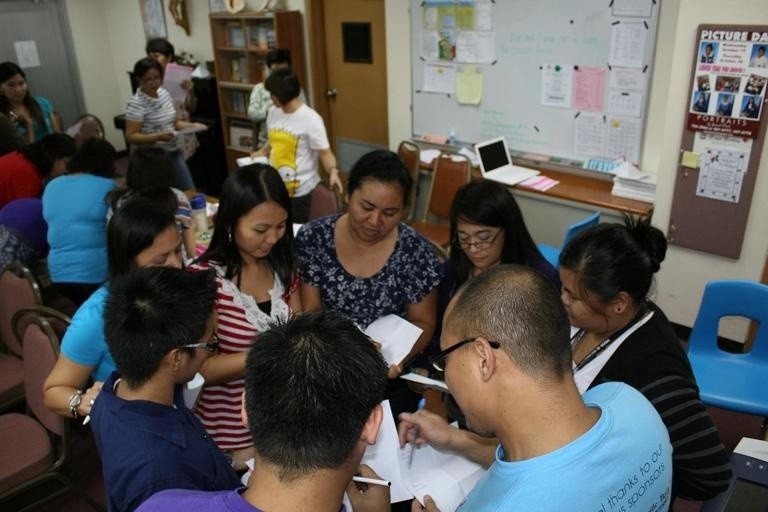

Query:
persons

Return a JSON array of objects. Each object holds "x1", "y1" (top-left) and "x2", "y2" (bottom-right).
[
  {"x1": 2, "y1": 38, "x2": 345, "y2": 512},
  {"x1": 558, "y1": 222, "x2": 732, "y2": 511},
  {"x1": 407, "y1": 177, "x2": 560, "y2": 394},
  {"x1": 137, "y1": 309, "x2": 393, "y2": 512},
  {"x1": 742, "y1": 97, "x2": 758, "y2": 118},
  {"x1": 397, "y1": 264, "x2": 673, "y2": 511},
  {"x1": 292, "y1": 148, "x2": 441, "y2": 379},
  {"x1": 749, "y1": 47, "x2": 768, "y2": 68}
]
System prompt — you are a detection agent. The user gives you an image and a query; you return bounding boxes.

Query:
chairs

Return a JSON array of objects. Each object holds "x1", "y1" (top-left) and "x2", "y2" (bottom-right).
[
  {"x1": 687, "y1": 278, "x2": 768, "y2": 417},
  {"x1": 73, "y1": 113, "x2": 105, "y2": 141},
  {"x1": 536, "y1": 210, "x2": 600, "y2": 271},
  {"x1": 0, "y1": 264, "x2": 107, "y2": 511},
  {"x1": 309, "y1": 141, "x2": 472, "y2": 262}
]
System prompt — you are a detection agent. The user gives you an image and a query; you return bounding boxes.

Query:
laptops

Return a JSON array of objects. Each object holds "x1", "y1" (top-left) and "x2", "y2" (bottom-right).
[{"x1": 474, "y1": 137, "x2": 541, "y2": 187}]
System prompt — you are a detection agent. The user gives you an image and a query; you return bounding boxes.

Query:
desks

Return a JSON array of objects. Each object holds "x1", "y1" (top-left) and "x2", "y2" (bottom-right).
[
  {"x1": 184, "y1": 190, "x2": 220, "y2": 229},
  {"x1": 416, "y1": 149, "x2": 653, "y2": 228}
]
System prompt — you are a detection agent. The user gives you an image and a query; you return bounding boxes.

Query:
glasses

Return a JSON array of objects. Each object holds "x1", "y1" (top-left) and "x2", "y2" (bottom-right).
[
  {"x1": 430, "y1": 337, "x2": 501, "y2": 373},
  {"x1": 450, "y1": 230, "x2": 502, "y2": 250},
  {"x1": 170, "y1": 331, "x2": 220, "y2": 353}
]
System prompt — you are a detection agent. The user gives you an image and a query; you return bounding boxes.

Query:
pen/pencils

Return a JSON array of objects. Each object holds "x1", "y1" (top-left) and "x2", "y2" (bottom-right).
[
  {"x1": 409, "y1": 398, "x2": 426, "y2": 470},
  {"x1": 9, "y1": 111, "x2": 17, "y2": 118},
  {"x1": 351, "y1": 476, "x2": 391, "y2": 487},
  {"x1": 82, "y1": 414, "x2": 92, "y2": 425}
]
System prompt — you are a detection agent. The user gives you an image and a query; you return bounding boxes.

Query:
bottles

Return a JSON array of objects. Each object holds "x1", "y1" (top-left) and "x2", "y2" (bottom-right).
[{"x1": 191, "y1": 195, "x2": 209, "y2": 232}]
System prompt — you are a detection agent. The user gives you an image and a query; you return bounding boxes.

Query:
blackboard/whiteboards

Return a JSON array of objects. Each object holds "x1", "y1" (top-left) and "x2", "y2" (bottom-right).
[{"x1": 409, "y1": 0, "x2": 662, "y2": 176}]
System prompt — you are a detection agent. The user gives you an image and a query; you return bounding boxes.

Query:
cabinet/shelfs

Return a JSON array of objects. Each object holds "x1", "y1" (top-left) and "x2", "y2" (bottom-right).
[{"x1": 209, "y1": 10, "x2": 310, "y2": 173}]
